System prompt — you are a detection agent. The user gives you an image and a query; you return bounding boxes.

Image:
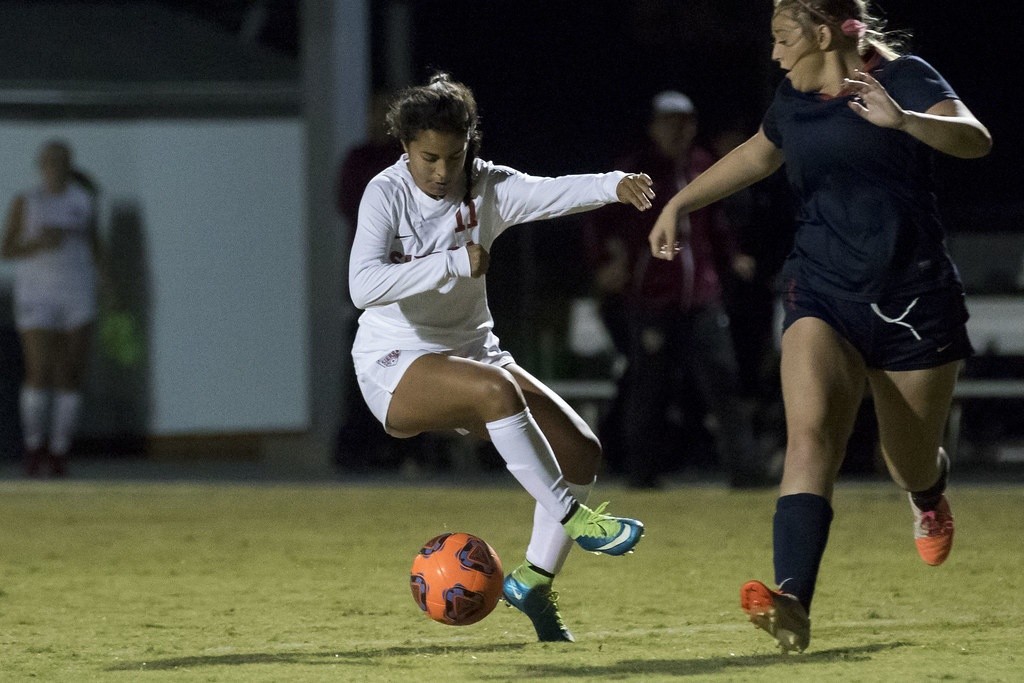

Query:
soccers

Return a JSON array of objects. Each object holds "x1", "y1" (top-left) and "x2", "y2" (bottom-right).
[{"x1": 409, "y1": 532, "x2": 507, "y2": 628}]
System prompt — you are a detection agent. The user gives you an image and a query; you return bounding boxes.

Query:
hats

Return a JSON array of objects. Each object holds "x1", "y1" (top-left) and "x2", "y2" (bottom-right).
[{"x1": 650, "y1": 91, "x2": 695, "y2": 117}]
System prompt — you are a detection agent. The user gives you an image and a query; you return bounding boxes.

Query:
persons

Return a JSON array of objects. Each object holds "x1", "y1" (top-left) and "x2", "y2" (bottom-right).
[
  {"x1": 588, "y1": 89, "x2": 778, "y2": 491},
  {"x1": 349, "y1": 79, "x2": 656, "y2": 640},
  {"x1": 647, "y1": 1, "x2": 993, "y2": 654},
  {"x1": 1, "y1": 140, "x2": 102, "y2": 477}
]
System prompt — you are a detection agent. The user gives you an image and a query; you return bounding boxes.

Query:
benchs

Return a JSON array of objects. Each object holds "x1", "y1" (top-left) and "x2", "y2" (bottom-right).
[{"x1": 539, "y1": 381, "x2": 1024, "y2": 476}]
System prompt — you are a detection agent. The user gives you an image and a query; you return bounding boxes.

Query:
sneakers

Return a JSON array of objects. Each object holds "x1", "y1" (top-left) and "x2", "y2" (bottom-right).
[
  {"x1": 560, "y1": 501, "x2": 644, "y2": 556},
  {"x1": 741, "y1": 581, "x2": 810, "y2": 653},
  {"x1": 909, "y1": 490, "x2": 954, "y2": 566},
  {"x1": 501, "y1": 559, "x2": 575, "y2": 643}
]
